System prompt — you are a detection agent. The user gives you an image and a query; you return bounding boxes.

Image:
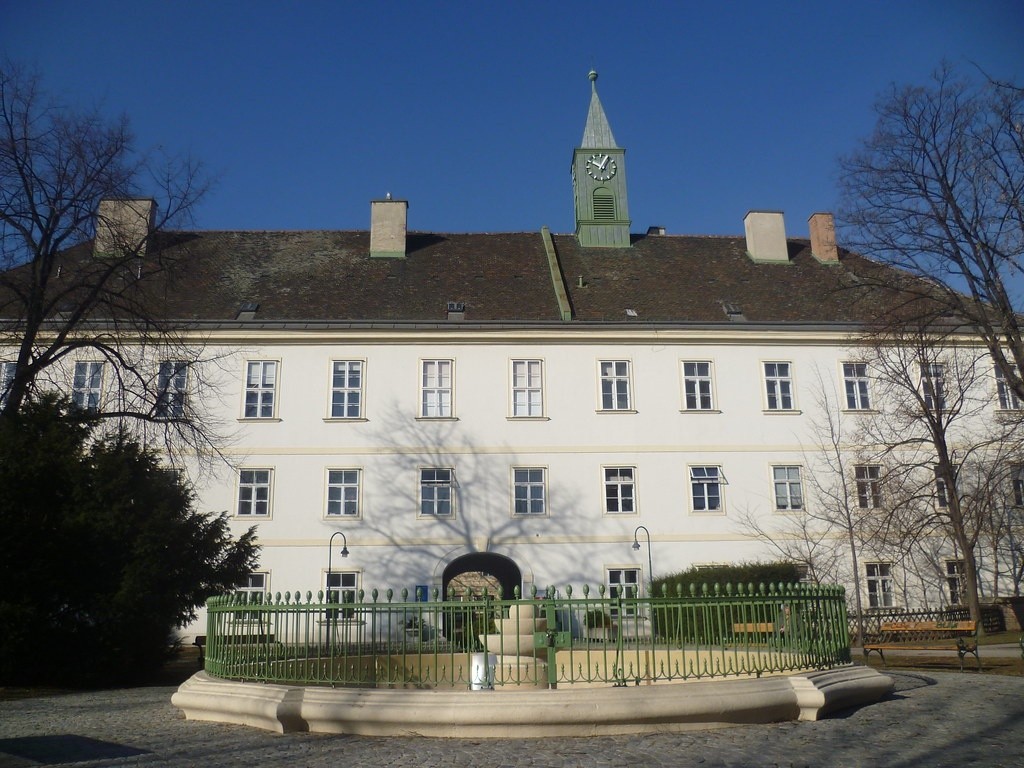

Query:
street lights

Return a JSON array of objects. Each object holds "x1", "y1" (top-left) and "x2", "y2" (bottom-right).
[
  {"x1": 632, "y1": 526, "x2": 654, "y2": 585},
  {"x1": 325, "y1": 531, "x2": 350, "y2": 657}
]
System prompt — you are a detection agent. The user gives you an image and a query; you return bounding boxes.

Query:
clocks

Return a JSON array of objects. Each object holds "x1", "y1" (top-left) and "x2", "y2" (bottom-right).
[{"x1": 586, "y1": 154, "x2": 617, "y2": 181}]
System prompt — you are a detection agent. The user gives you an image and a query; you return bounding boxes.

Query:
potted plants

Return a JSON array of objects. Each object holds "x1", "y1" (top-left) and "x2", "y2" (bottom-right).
[
  {"x1": 583, "y1": 610, "x2": 617, "y2": 641},
  {"x1": 400, "y1": 617, "x2": 428, "y2": 644}
]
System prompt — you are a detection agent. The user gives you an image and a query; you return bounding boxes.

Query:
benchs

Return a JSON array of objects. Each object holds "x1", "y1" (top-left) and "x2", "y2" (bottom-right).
[
  {"x1": 733, "y1": 623, "x2": 773, "y2": 644},
  {"x1": 194, "y1": 634, "x2": 284, "y2": 664},
  {"x1": 864, "y1": 620, "x2": 985, "y2": 674}
]
